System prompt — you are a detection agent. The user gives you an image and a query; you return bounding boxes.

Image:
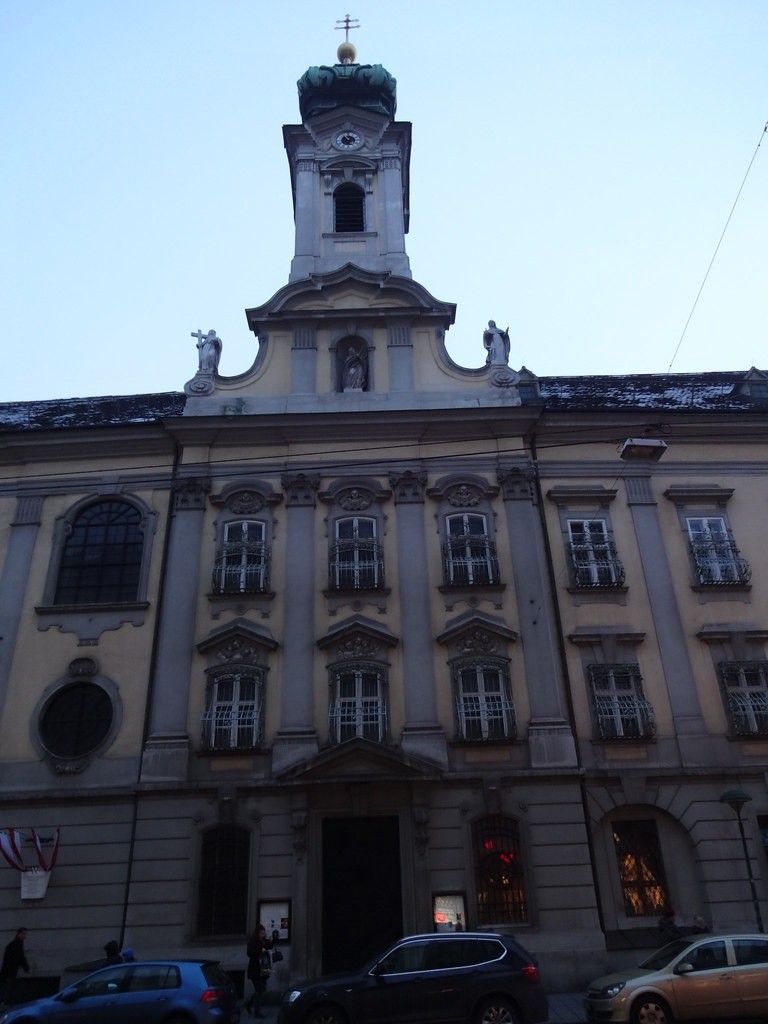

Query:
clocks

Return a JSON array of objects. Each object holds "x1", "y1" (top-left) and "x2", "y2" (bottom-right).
[{"x1": 334, "y1": 130, "x2": 361, "y2": 150}]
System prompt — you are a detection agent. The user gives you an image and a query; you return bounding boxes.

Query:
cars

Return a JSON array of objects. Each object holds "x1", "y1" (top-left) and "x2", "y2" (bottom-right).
[
  {"x1": 583, "y1": 931, "x2": 768, "y2": 1024},
  {"x1": 0, "y1": 959, "x2": 239, "y2": 1024}
]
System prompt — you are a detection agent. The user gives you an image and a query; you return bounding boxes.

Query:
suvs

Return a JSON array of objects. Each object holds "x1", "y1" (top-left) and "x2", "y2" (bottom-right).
[{"x1": 274, "y1": 931, "x2": 551, "y2": 1024}]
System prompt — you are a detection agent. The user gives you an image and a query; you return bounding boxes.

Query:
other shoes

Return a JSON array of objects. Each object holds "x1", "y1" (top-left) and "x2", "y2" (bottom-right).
[
  {"x1": 243, "y1": 1001, "x2": 253, "y2": 1014},
  {"x1": 254, "y1": 1011, "x2": 265, "y2": 1019}
]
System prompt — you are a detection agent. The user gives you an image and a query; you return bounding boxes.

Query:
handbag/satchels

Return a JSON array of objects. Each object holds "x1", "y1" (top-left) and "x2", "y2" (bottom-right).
[{"x1": 271, "y1": 947, "x2": 284, "y2": 963}]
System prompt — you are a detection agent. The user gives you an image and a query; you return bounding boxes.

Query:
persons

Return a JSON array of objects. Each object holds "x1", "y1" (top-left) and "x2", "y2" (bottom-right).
[
  {"x1": 196, "y1": 329, "x2": 223, "y2": 376},
  {"x1": 341, "y1": 347, "x2": 365, "y2": 389},
  {"x1": 483, "y1": 319, "x2": 511, "y2": 364},
  {"x1": 691, "y1": 916, "x2": 710, "y2": 935},
  {"x1": 104, "y1": 940, "x2": 134, "y2": 966},
  {"x1": 659, "y1": 909, "x2": 681, "y2": 948},
  {"x1": 0, "y1": 927, "x2": 30, "y2": 1006},
  {"x1": 243, "y1": 925, "x2": 278, "y2": 1019}
]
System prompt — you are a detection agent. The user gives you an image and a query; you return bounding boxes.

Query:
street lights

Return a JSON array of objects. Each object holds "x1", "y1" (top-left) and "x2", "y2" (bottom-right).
[{"x1": 719, "y1": 790, "x2": 765, "y2": 934}]
